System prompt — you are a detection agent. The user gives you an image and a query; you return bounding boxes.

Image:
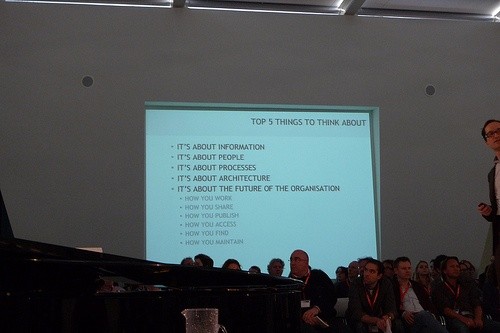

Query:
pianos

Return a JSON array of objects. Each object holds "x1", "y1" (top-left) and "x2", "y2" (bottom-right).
[{"x1": 0, "y1": 190, "x2": 309, "y2": 333}]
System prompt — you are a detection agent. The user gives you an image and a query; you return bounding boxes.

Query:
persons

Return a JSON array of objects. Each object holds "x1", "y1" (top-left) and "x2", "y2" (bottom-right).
[
  {"x1": 181, "y1": 248, "x2": 495, "y2": 333},
  {"x1": 475, "y1": 118, "x2": 500, "y2": 254}
]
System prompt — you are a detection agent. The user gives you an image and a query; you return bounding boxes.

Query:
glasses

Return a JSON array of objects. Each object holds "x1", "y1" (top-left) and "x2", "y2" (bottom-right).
[{"x1": 484, "y1": 129, "x2": 500, "y2": 138}]
